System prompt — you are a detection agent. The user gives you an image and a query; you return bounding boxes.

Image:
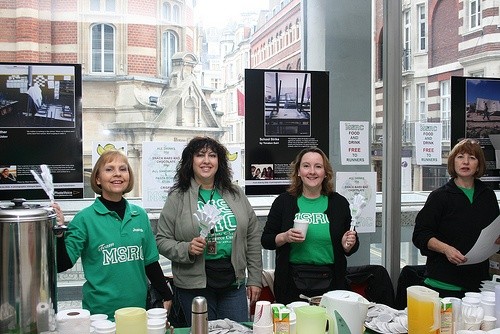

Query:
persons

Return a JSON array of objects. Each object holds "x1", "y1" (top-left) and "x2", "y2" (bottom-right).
[
  {"x1": 156, "y1": 137, "x2": 263, "y2": 328},
  {"x1": 1, "y1": 169, "x2": 16, "y2": 181},
  {"x1": 251, "y1": 165, "x2": 273, "y2": 179},
  {"x1": 412, "y1": 139, "x2": 500, "y2": 298},
  {"x1": 52, "y1": 151, "x2": 173, "y2": 323},
  {"x1": 260, "y1": 148, "x2": 360, "y2": 306}
]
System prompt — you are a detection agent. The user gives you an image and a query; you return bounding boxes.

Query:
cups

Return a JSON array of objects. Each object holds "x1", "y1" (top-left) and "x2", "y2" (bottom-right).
[
  {"x1": 443, "y1": 292, "x2": 500, "y2": 333},
  {"x1": 252, "y1": 300, "x2": 310, "y2": 334},
  {"x1": 294, "y1": 219, "x2": 309, "y2": 240},
  {"x1": 90, "y1": 306, "x2": 168, "y2": 334}
]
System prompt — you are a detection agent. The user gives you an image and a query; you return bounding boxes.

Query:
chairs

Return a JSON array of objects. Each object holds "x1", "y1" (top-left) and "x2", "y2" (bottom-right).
[
  {"x1": 333, "y1": 263, "x2": 394, "y2": 307},
  {"x1": 396, "y1": 265, "x2": 425, "y2": 309}
]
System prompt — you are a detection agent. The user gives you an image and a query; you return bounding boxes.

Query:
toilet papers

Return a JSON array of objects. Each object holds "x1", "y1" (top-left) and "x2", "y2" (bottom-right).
[{"x1": 56, "y1": 309, "x2": 91, "y2": 334}]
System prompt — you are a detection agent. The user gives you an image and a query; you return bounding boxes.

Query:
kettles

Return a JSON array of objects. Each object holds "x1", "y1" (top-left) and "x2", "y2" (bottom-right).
[{"x1": 319, "y1": 289, "x2": 370, "y2": 334}]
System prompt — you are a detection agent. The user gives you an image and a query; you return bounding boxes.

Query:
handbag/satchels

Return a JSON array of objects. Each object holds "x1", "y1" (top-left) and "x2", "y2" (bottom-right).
[
  {"x1": 292, "y1": 264, "x2": 333, "y2": 290},
  {"x1": 206, "y1": 259, "x2": 236, "y2": 288}
]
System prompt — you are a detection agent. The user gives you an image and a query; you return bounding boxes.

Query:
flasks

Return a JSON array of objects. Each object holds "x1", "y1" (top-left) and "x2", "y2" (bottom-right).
[{"x1": 191, "y1": 296, "x2": 208, "y2": 333}]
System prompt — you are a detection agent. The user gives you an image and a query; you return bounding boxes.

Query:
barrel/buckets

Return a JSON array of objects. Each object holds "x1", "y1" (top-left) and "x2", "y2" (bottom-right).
[{"x1": 0, "y1": 198, "x2": 67, "y2": 334}]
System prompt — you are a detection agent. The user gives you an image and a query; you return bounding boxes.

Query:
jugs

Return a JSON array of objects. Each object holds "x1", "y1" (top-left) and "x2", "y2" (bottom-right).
[
  {"x1": 293, "y1": 306, "x2": 334, "y2": 334},
  {"x1": 406, "y1": 284, "x2": 439, "y2": 334}
]
